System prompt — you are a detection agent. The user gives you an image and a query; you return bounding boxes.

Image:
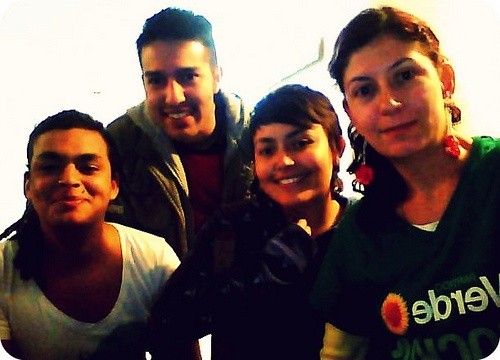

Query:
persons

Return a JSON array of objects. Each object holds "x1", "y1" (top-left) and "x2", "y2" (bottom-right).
[
  {"x1": 0, "y1": 110, "x2": 202, "y2": 360},
  {"x1": 104, "y1": 5, "x2": 500, "y2": 360}
]
